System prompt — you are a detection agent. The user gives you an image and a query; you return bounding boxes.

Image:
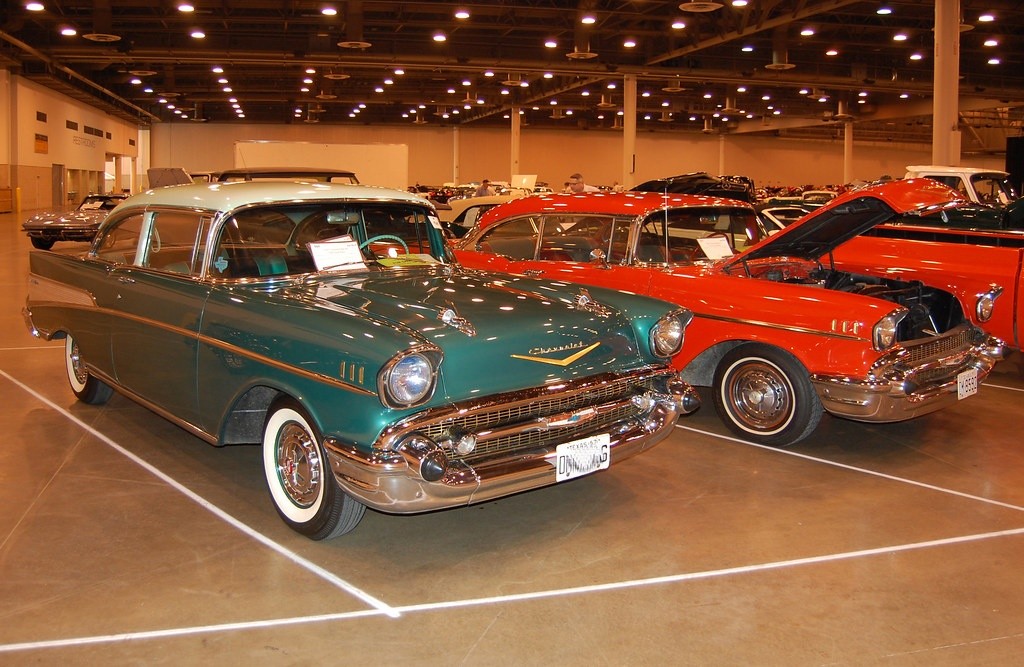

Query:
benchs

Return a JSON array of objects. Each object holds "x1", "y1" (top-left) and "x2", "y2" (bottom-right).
[{"x1": 478, "y1": 237, "x2": 598, "y2": 262}]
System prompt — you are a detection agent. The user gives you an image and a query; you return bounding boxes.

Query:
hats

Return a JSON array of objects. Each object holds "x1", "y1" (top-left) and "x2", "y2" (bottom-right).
[{"x1": 482, "y1": 179, "x2": 490, "y2": 183}]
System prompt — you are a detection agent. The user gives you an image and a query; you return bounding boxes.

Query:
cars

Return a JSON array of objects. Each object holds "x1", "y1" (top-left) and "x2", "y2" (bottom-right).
[
  {"x1": 146, "y1": 166, "x2": 223, "y2": 244},
  {"x1": 219, "y1": 167, "x2": 362, "y2": 246},
  {"x1": 21, "y1": 181, "x2": 703, "y2": 542},
  {"x1": 406, "y1": 166, "x2": 1023, "y2": 354},
  {"x1": 369, "y1": 178, "x2": 1011, "y2": 447},
  {"x1": 20, "y1": 193, "x2": 145, "y2": 250}
]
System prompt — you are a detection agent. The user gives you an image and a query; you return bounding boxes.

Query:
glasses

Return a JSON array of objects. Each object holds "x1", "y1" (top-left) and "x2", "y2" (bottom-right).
[{"x1": 569, "y1": 183, "x2": 579, "y2": 185}]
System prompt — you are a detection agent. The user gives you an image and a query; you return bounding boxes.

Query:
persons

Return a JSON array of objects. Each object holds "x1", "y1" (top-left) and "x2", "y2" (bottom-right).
[
  {"x1": 416, "y1": 182, "x2": 419, "y2": 186},
  {"x1": 539, "y1": 183, "x2": 553, "y2": 191},
  {"x1": 476, "y1": 180, "x2": 496, "y2": 196},
  {"x1": 564, "y1": 182, "x2": 572, "y2": 193},
  {"x1": 613, "y1": 181, "x2": 621, "y2": 191},
  {"x1": 570, "y1": 173, "x2": 600, "y2": 223}
]
